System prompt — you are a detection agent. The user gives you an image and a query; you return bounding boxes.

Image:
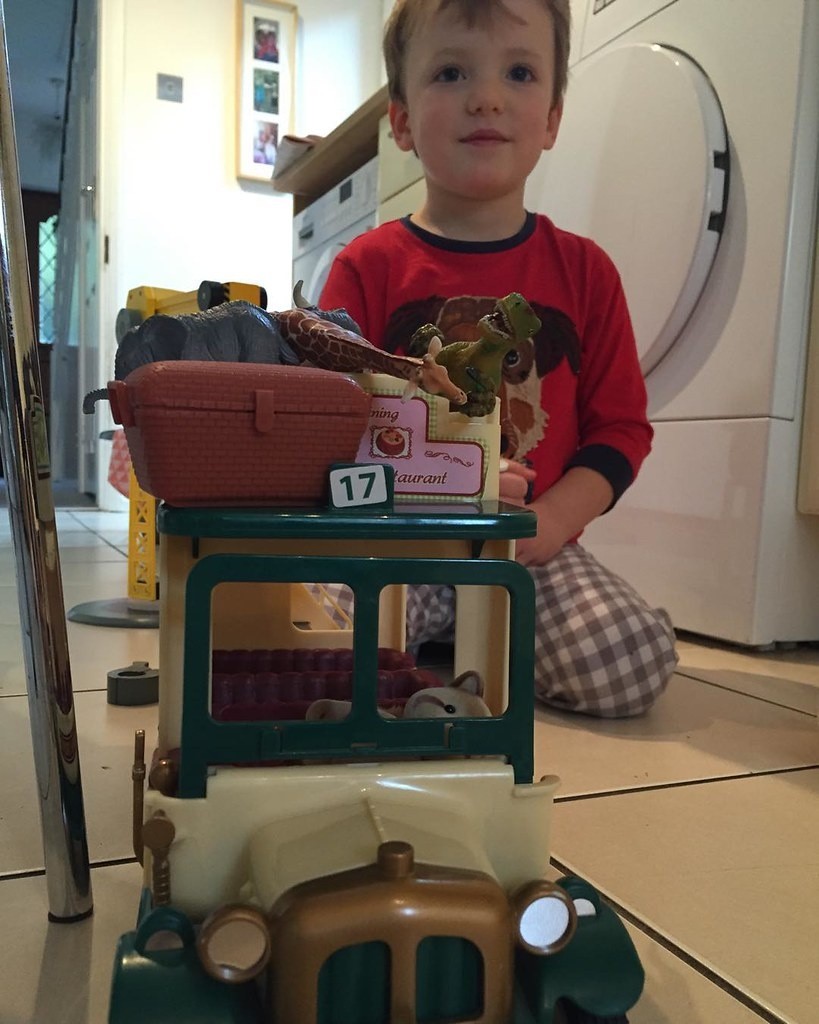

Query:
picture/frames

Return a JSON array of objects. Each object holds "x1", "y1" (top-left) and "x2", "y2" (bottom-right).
[{"x1": 230, "y1": 0, "x2": 298, "y2": 184}]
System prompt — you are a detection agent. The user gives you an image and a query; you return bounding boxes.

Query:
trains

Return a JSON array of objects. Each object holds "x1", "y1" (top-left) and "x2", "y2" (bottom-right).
[{"x1": 104, "y1": 499, "x2": 646, "y2": 1024}]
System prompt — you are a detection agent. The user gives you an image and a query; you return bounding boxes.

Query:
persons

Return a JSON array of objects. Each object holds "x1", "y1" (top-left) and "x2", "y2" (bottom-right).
[
  {"x1": 316, "y1": 0, "x2": 681, "y2": 719},
  {"x1": 254, "y1": 29, "x2": 278, "y2": 165}
]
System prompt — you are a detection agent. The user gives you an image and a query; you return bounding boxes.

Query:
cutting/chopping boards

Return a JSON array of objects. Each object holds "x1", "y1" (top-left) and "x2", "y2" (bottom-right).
[{"x1": 273, "y1": 82, "x2": 391, "y2": 201}]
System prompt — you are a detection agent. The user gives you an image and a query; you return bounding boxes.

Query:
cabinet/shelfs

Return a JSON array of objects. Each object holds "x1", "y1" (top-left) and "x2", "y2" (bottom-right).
[{"x1": 374, "y1": 112, "x2": 426, "y2": 226}]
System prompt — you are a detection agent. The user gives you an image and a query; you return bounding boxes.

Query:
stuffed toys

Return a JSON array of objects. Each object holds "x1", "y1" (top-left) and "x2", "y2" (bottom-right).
[{"x1": 305, "y1": 667, "x2": 496, "y2": 718}]
